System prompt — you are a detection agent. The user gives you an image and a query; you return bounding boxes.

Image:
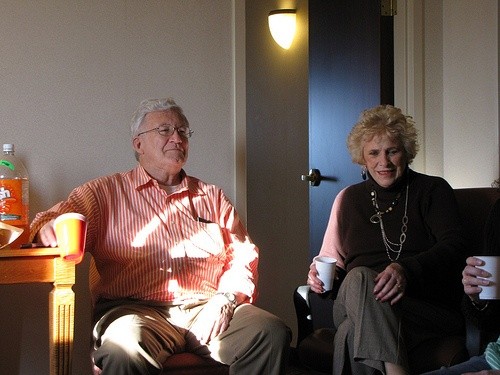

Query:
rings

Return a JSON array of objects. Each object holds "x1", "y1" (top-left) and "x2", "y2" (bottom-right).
[{"x1": 398, "y1": 284, "x2": 403, "y2": 289}]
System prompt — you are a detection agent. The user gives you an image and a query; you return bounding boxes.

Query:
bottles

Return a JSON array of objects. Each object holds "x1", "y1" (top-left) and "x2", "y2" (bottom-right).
[{"x1": 0, "y1": 144, "x2": 31, "y2": 249}]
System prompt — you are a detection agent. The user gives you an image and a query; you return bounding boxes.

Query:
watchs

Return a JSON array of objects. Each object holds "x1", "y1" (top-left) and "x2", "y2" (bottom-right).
[{"x1": 216, "y1": 290, "x2": 238, "y2": 308}]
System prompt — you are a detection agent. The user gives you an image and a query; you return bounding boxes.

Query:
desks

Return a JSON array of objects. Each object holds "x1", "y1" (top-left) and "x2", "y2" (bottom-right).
[{"x1": 0, "y1": 245, "x2": 75, "y2": 375}]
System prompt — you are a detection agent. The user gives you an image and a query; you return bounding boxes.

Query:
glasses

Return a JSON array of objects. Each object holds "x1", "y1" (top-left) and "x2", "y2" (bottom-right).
[{"x1": 137, "y1": 124, "x2": 194, "y2": 140}]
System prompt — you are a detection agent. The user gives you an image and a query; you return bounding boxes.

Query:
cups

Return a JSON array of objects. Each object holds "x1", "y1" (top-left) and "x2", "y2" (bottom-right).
[
  {"x1": 313, "y1": 257, "x2": 337, "y2": 291},
  {"x1": 54, "y1": 212, "x2": 88, "y2": 264},
  {"x1": 473, "y1": 256, "x2": 500, "y2": 299}
]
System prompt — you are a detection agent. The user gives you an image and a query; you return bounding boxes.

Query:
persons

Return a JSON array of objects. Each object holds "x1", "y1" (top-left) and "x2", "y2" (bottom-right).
[
  {"x1": 307, "y1": 104, "x2": 467, "y2": 375},
  {"x1": 424, "y1": 195, "x2": 500, "y2": 375},
  {"x1": 30, "y1": 96, "x2": 294, "y2": 375}
]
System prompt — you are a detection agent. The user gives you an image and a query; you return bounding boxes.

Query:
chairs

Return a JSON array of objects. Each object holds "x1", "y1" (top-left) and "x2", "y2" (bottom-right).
[
  {"x1": 90, "y1": 320, "x2": 230, "y2": 375},
  {"x1": 293, "y1": 187, "x2": 500, "y2": 375}
]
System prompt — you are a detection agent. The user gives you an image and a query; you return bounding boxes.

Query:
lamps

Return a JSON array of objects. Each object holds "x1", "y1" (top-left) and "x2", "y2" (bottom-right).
[{"x1": 268, "y1": 9, "x2": 297, "y2": 49}]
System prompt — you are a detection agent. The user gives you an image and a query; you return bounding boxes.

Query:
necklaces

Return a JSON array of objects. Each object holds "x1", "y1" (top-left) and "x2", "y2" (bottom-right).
[{"x1": 370, "y1": 178, "x2": 409, "y2": 261}]
